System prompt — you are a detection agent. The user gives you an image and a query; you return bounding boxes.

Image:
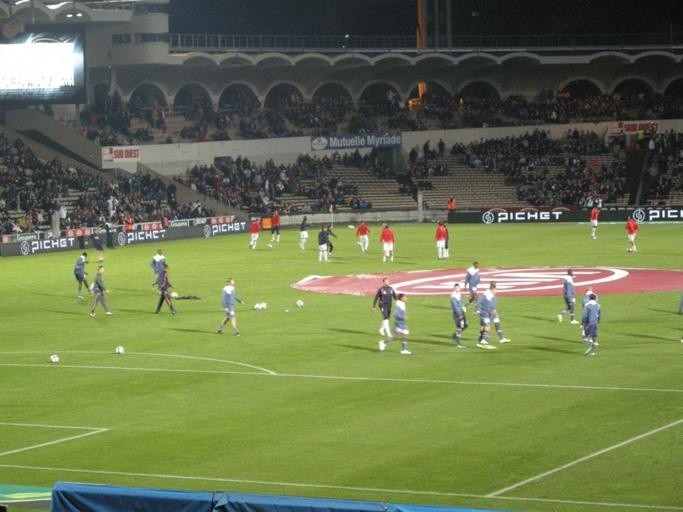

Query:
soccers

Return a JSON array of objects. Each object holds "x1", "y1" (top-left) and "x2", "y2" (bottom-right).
[
  {"x1": 170, "y1": 292, "x2": 177, "y2": 296},
  {"x1": 51, "y1": 355, "x2": 59, "y2": 361},
  {"x1": 116, "y1": 345, "x2": 124, "y2": 354},
  {"x1": 268, "y1": 244, "x2": 272, "y2": 247},
  {"x1": 296, "y1": 301, "x2": 303, "y2": 308},
  {"x1": 254, "y1": 303, "x2": 266, "y2": 309}
]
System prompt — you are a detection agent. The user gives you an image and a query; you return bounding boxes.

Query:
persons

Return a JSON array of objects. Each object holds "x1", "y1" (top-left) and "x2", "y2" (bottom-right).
[
  {"x1": 581, "y1": 287, "x2": 599, "y2": 353},
  {"x1": 378, "y1": 293, "x2": 411, "y2": 354},
  {"x1": 217, "y1": 277, "x2": 245, "y2": 336},
  {"x1": 449, "y1": 281, "x2": 511, "y2": 347},
  {"x1": 154, "y1": 263, "x2": 177, "y2": 314},
  {"x1": 88, "y1": 265, "x2": 113, "y2": 317},
  {"x1": 150, "y1": 249, "x2": 171, "y2": 287},
  {"x1": 74, "y1": 252, "x2": 94, "y2": 299},
  {"x1": 556, "y1": 268, "x2": 580, "y2": 324},
  {"x1": 371, "y1": 277, "x2": 398, "y2": 337},
  {"x1": 461, "y1": 261, "x2": 481, "y2": 313},
  {"x1": 678, "y1": 292, "x2": 683, "y2": 344},
  {"x1": 1, "y1": 69, "x2": 683, "y2": 261}
]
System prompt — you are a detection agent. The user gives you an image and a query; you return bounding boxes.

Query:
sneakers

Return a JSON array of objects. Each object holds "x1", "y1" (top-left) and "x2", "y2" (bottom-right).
[
  {"x1": 557, "y1": 315, "x2": 562, "y2": 323},
  {"x1": 379, "y1": 341, "x2": 384, "y2": 351},
  {"x1": 499, "y1": 338, "x2": 510, "y2": 343},
  {"x1": 400, "y1": 351, "x2": 410, "y2": 355},
  {"x1": 570, "y1": 320, "x2": 578, "y2": 325}
]
